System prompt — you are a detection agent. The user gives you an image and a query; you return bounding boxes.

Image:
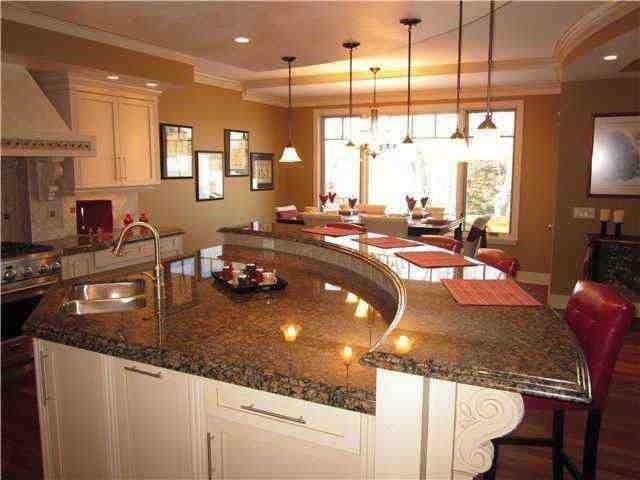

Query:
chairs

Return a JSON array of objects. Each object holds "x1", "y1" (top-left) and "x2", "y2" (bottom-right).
[
  {"x1": 482, "y1": 279, "x2": 635, "y2": 480},
  {"x1": 275, "y1": 200, "x2": 519, "y2": 276}
]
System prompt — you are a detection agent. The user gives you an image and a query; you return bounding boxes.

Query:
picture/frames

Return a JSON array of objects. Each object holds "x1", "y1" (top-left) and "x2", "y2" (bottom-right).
[
  {"x1": 159, "y1": 122, "x2": 274, "y2": 201},
  {"x1": 585, "y1": 111, "x2": 640, "y2": 198}
]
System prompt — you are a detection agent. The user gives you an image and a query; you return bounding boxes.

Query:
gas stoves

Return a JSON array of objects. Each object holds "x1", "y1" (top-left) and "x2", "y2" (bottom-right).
[{"x1": 0, "y1": 240, "x2": 61, "y2": 285}]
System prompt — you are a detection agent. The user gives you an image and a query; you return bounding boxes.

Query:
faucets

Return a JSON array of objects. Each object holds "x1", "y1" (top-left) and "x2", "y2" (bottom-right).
[
  {"x1": 109, "y1": 222, "x2": 167, "y2": 300},
  {"x1": 113, "y1": 300, "x2": 166, "y2": 352}
]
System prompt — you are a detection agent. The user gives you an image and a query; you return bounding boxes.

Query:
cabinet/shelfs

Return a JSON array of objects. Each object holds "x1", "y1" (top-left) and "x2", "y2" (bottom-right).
[
  {"x1": 31, "y1": 335, "x2": 523, "y2": 480},
  {"x1": 573, "y1": 228, "x2": 640, "y2": 317},
  {"x1": 43, "y1": 76, "x2": 163, "y2": 195},
  {"x1": 61, "y1": 232, "x2": 187, "y2": 285}
]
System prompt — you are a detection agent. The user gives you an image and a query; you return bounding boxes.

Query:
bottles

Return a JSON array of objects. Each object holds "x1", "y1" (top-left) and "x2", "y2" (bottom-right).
[
  {"x1": 138, "y1": 211, "x2": 150, "y2": 237},
  {"x1": 123, "y1": 213, "x2": 135, "y2": 240}
]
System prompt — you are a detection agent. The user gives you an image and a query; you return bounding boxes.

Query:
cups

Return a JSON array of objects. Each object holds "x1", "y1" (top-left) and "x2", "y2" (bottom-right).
[{"x1": 216, "y1": 262, "x2": 278, "y2": 288}]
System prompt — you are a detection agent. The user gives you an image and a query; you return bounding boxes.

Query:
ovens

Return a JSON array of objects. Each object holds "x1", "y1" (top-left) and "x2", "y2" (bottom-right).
[{"x1": 1, "y1": 272, "x2": 61, "y2": 343}]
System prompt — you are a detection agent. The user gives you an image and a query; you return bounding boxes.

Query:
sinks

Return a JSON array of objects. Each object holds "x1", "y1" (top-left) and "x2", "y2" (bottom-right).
[
  {"x1": 54, "y1": 295, "x2": 148, "y2": 318},
  {"x1": 60, "y1": 275, "x2": 148, "y2": 299}
]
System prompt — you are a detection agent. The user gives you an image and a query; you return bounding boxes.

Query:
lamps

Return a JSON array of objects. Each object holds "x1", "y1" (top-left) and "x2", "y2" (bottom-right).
[{"x1": 278, "y1": 1, "x2": 503, "y2": 164}]
